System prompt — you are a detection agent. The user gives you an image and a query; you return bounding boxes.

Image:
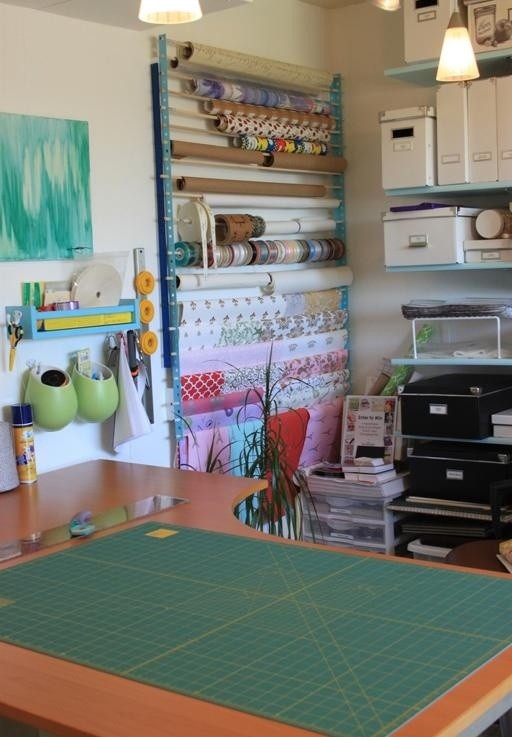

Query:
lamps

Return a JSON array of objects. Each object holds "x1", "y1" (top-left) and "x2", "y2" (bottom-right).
[
  {"x1": 436, "y1": 0, "x2": 480, "y2": 83},
  {"x1": 138, "y1": 0, "x2": 202, "y2": 24}
]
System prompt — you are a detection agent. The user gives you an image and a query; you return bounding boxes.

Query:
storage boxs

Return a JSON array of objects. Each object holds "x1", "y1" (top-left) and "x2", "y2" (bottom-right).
[
  {"x1": 301, "y1": 374, "x2": 512, "y2": 561},
  {"x1": 379, "y1": 0, "x2": 512, "y2": 266}
]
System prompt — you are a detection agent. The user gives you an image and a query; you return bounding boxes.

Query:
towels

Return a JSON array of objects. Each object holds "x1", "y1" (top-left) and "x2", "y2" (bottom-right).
[{"x1": 114, "y1": 338, "x2": 152, "y2": 452}]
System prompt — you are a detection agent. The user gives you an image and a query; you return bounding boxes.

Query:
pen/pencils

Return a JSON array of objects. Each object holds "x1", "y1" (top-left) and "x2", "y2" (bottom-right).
[{"x1": 91, "y1": 371, "x2": 105, "y2": 381}]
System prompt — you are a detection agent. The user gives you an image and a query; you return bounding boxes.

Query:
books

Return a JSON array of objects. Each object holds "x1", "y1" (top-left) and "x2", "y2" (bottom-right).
[
  {"x1": 386, "y1": 492, "x2": 511, "y2": 549},
  {"x1": 342, "y1": 462, "x2": 394, "y2": 474},
  {"x1": 345, "y1": 470, "x2": 397, "y2": 483}
]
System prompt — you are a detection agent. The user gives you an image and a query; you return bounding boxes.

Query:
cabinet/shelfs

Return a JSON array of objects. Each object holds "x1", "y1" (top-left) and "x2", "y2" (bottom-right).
[{"x1": 385, "y1": 48, "x2": 512, "y2": 365}]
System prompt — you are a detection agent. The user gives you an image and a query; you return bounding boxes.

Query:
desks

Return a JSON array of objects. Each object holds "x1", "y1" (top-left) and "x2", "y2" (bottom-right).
[
  {"x1": 447, "y1": 538, "x2": 512, "y2": 573},
  {"x1": 1, "y1": 459, "x2": 511, "y2": 737}
]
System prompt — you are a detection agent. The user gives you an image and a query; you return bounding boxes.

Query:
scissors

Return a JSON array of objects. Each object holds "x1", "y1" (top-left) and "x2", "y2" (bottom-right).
[{"x1": 7, "y1": 324, "x2": 24, "y2": 371}]
